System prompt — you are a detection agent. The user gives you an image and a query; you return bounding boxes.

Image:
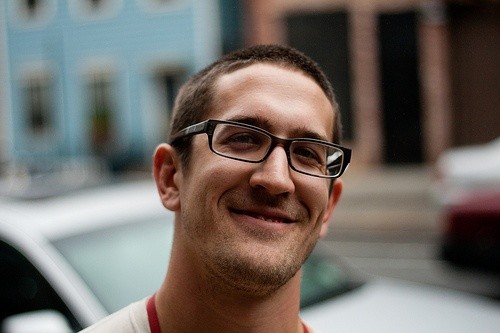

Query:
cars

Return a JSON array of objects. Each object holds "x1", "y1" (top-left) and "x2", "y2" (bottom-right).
[
  {"x1": 439, "y1": 183, "x2": 500, "y2": 275},
  {"x1": 0, "y1": 175, "x2": 500, "y2": 333}
]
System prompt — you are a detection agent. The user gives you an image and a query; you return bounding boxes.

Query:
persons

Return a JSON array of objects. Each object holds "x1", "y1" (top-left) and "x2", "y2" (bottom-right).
[{"x1": 78, "y1": 43, "x2": 353, "y2": 333}]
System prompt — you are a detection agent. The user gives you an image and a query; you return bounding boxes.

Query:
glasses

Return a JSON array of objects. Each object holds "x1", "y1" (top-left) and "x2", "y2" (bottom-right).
[{"x1": 170, "y1": 120, "x2": 352, "y2": 178}]
